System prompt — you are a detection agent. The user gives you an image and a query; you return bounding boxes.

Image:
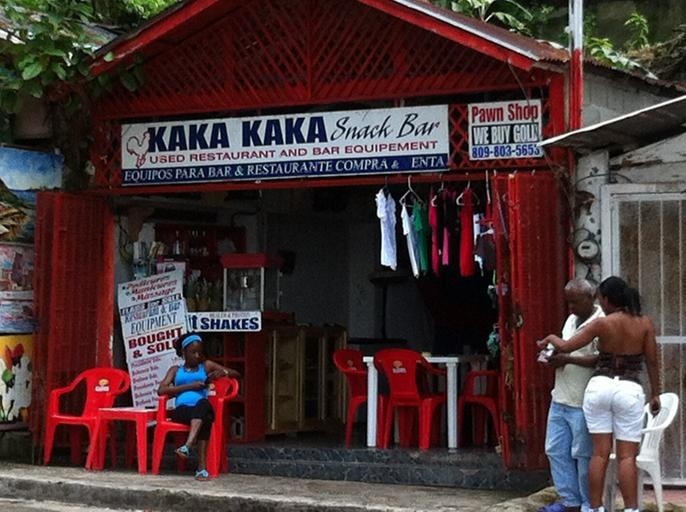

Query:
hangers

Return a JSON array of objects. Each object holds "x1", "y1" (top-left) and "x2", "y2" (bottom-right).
[{"x1": 372, "y1": 171, "x2": 536, "y2": 206}]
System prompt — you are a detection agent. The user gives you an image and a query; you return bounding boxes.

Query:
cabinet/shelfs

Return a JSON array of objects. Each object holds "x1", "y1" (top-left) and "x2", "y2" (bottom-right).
[
  {"x1": 271, "y1": 325, "x2": 345, "y2": 432},
  {"x1": 204, "y1": 323, "x2": 270, "y2": 442}
]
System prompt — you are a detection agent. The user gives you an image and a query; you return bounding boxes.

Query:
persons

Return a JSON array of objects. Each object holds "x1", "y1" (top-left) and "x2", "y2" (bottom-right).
[
  {"x1": 155, "y1": 331, "x2": 242, "y2": 482},
  {"x1": 535, "y1": 274, "x2": 662, "y2": 511},
  {"x1": 534, "y1": 276, "x2": 607, "y2": 511}
]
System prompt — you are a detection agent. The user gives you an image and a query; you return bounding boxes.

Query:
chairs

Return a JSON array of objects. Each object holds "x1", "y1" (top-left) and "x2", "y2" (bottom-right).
[
  {"x1": 602, "y1": 391, "x2": 680, "y2": 512},
  {"x1": 333, "y1": 345, "x2": 498, "y2": 459},
  {"x1": 150, "y1": 376, "x2": 238, "y2": 476},
  {"x1": 43, "y1": 366, "x2": 131, "y2": 474}
]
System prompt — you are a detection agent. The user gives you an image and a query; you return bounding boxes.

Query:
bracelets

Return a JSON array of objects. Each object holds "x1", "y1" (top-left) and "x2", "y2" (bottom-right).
[{"x1": 219, "y1": 367, "x2": 229, "y2": 376}]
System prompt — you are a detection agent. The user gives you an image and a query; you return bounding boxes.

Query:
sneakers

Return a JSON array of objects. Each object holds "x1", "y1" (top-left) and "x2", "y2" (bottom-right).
[
  {"x1": 174, "y1": 444, "x2": 192, "y2": 460},
  {"x1": 194, "y1": 469, "x2": 214, "y2": 481},
  {"x1": 537, "y1": 503, "x2": 583, "y2": 512}
]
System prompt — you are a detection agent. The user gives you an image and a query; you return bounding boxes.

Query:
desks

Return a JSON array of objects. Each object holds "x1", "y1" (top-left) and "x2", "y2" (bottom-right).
[{"x1": 84, "y1": 407, "x2": 155, "y2": 474}]
